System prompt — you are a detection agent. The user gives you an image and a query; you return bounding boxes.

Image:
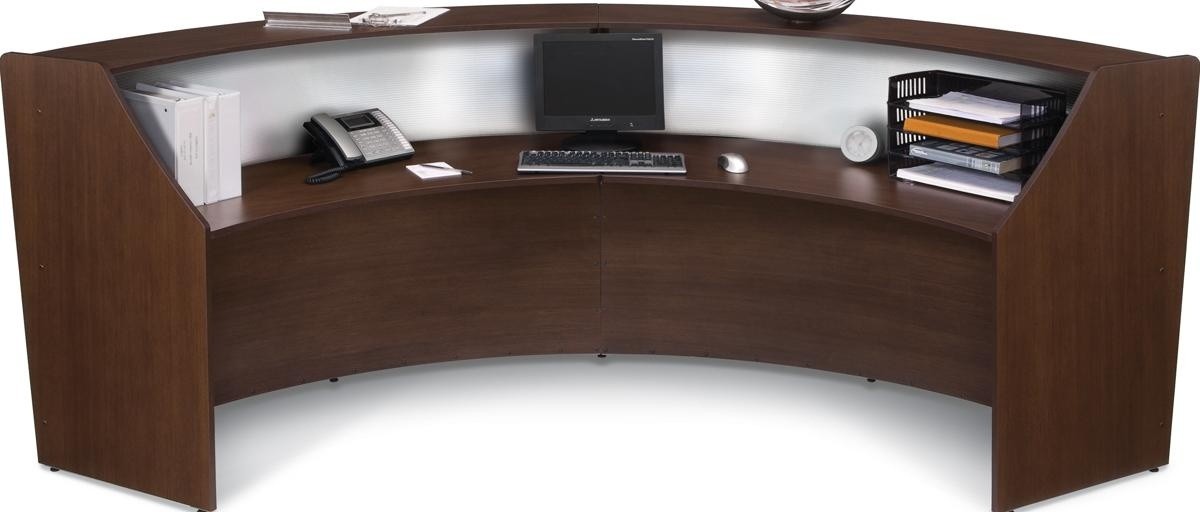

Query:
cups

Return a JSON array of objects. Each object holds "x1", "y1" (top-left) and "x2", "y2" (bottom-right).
[{"x1": 841, "y1": 126, "x2": 882, "y2": 165}]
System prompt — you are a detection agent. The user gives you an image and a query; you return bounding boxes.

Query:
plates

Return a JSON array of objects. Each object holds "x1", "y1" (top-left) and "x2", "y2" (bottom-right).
[{"x1": 754, "y1": 0, "x2": 856, "y2": 25}]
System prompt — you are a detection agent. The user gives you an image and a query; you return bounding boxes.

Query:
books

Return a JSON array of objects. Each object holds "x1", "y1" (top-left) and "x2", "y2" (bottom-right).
[
  {"x1": 897, "y1": 91, "x2": 1048, "y2": 203},
  {"x1": 120, "y1": 79, "x2": 242, "y2": 207}
]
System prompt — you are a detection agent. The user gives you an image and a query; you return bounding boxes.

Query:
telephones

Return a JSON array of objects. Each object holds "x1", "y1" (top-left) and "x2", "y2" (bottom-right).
[{"x1": 302, "y1": 107, "x2": 416, "y2": 170}]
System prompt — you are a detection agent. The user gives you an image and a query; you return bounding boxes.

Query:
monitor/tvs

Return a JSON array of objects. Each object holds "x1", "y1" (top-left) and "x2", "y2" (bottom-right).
[{"x1": 534, "y1": 32, "x2": 664, "y2": 152}]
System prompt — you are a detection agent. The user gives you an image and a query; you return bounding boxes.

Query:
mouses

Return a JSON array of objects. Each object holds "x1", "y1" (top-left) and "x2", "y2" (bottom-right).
[{"x1": 719, "y1": 152, "x2": 748, "y2": 173}]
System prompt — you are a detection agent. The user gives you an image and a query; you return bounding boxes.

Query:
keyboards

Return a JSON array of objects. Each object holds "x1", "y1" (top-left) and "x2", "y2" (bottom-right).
[{"x1": 517, "y1": 150, "x2": 687, "y2": 174}]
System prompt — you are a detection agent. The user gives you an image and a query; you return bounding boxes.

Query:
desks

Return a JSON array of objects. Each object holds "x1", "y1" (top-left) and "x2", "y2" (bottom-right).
[{"x1": 0, "y1": 4, "x2": 1179, "y2": 511}]
